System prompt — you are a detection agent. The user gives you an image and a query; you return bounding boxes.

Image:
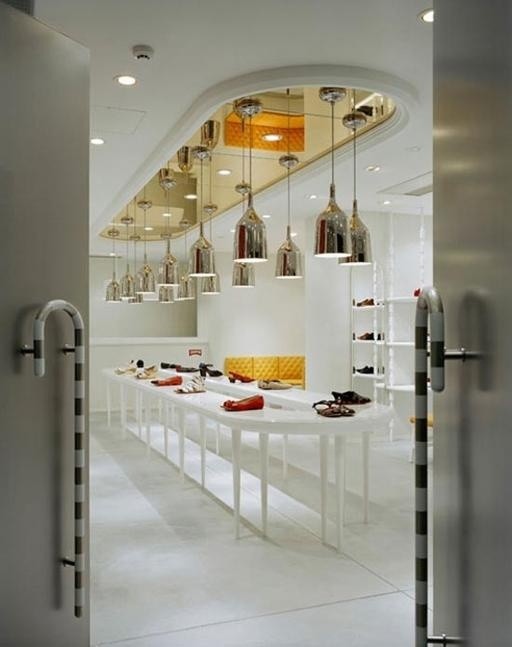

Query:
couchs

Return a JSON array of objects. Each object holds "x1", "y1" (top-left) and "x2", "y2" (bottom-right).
[
  {"x1": 224, "y1": 109, "x2": 305, "y2": 152},
  {"x1": 225, "y1": 355, "x2": 305, "y2": 389}
]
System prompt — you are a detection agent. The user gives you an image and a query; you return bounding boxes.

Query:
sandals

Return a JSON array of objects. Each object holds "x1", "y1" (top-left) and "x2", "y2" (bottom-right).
[
  {"x1": 313, "y1": 400, "x2": 353, "y2": 418},
  {"x1": 176, "y1": 376, "x2": 207, "y2": 392}
]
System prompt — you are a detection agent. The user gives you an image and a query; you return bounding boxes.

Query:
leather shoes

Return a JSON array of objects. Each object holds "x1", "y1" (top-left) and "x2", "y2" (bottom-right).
[
  {"x1": 357, "y1": 366, "x2": 384, "y2": 374},
  {"x1": 358, "y1": 299, "x2": 375, "y2": 305},
  {"x1": 358, "y1": 332, "x2": 385, "y2": 341},
  {"x1": 220, "y1": 395, "x2": 263, "y2": 410},
  {"x1": 176, "y1": 365, "x2": 199, "y2": 372},
  {"x1": 151, "y1": 377, "x2": 182, "y2": 385},
  {"x1": 161, "y1": 363, "x2": 182, "y2": 369}
]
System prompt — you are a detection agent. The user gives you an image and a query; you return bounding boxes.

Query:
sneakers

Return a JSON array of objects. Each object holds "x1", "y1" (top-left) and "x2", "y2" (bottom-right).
[{"x1": 258, "y1": 379, "x2": 292, "y2": 389}]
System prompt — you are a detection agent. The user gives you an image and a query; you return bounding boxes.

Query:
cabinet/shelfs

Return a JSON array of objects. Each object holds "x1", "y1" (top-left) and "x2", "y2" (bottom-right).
[{"x1": 350, "y1": 294, "x2": 432, "y2": 464}]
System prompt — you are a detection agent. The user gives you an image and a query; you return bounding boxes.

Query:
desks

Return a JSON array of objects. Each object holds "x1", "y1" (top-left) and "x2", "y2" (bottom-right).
[{"x1": 102, "y1": 362, "x2": 395, "y2": 554}]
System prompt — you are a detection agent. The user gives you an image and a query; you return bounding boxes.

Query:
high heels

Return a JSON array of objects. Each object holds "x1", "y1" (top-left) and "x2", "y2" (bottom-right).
[
  {"x1": 229, "y1": 372, "x2": 255, "y2": 383},
  {"x1": 199, "y1": 363, "x2": 223, "y2": 376},
  {"x1": 331, "y1": 391, "x2": 370, "y2": 404},
  {"x1": 136, "y1": 365, "x2": 158, "y2": 379}
]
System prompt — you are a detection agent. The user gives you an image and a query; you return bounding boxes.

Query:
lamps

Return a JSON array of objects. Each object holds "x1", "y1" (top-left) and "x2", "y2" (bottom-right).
[
  {"x1": 230, "y1": 182, "x2": 257, "y2": 289},
  {"x1": 186, "y1": 146, "x2": 216, "y2": 278},
  {"x1": 105, "y1": 198, "x2": 155, "y2": 304},
  {"x1": 198, "y1": 203, "x2": 223, "y2": 296},
  {"x1": 313, "y1": 87, "x2": 353, "y2": 259},
  {"x1": 156, "y1": 177, "x2": 182, "y2": 287},
  {"x1": 338, "y1": 112, "x2": 372, "y2": 267},
  {"x1": 274, "y1": 154, "x2": 306, "y2": 280},
  {"x1": 158, "y1": 232, "x2": 179, "y2": 304},
  {"x1": 232, "y1": 97, "x2": 268, "y2": 264}
]
